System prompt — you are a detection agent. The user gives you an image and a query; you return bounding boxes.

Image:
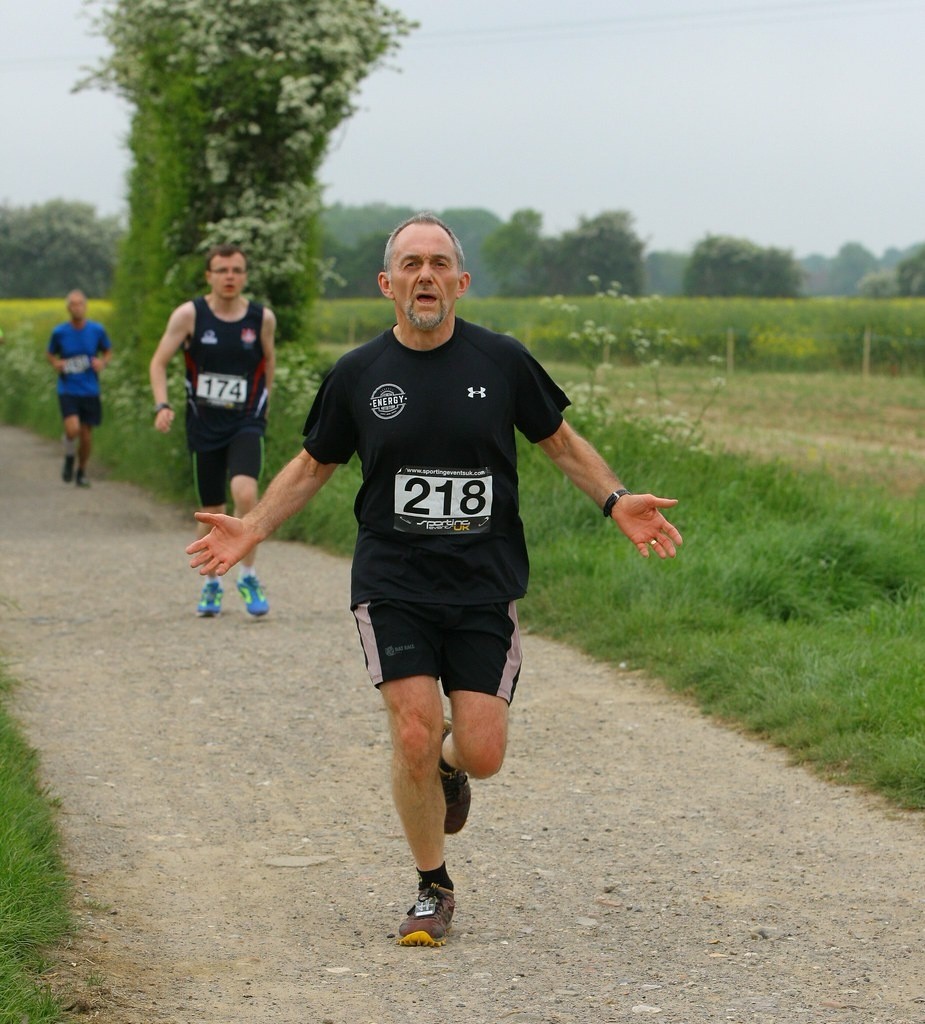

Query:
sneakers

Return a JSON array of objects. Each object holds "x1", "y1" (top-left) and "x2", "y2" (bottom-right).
[
  {"x1": 237, "y1": 573, "x2": 269, "y2": 615},
  {"x1": 436, "y1": 716, "x2": 471, "y2": 834},
  {"x1": 396, "y1": 883, "x2": 455, "y2": 947},
  {"x1": 196, "y1": 580, "x2": 224, "y2": 617}
]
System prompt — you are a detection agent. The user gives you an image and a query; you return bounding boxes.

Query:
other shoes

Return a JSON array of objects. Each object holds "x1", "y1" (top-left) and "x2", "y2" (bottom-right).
[
  {"x1": 62, "y1": 454, "x2": 74, "y2": 482},
  {"x1": 76, "y1": 471, "x2": 91, "y2": 488}
]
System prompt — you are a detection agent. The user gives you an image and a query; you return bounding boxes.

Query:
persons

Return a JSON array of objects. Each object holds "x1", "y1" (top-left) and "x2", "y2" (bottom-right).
[
  {"x1": 188, "y1": 211, "x2": 685, "y2": 945},
  {"x1": 45, "y1": 289, "x2": 113, "y2": 488},
  {"x1": 148, "y1": 234, "x2": 276, "y2": 616}
]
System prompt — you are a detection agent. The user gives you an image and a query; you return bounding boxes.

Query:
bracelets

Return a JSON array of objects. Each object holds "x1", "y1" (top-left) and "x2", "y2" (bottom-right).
[
  {"x1": 155, "y1": 403, "x2": 171, "y2": 415},
  {"x1": 602, "y1": 489, "x2": 632, "y2": 519}
]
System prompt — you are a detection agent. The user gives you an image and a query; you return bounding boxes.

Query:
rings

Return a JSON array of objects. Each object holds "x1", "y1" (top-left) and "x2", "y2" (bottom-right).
[{"x1": 651, "y1": 540, "x2": 657, "y2": 546}]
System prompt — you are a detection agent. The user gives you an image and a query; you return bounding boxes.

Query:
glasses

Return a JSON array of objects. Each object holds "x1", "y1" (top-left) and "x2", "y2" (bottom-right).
[{"x1": 210, "y1": 267, "x2": 247, "y2": 274}]
